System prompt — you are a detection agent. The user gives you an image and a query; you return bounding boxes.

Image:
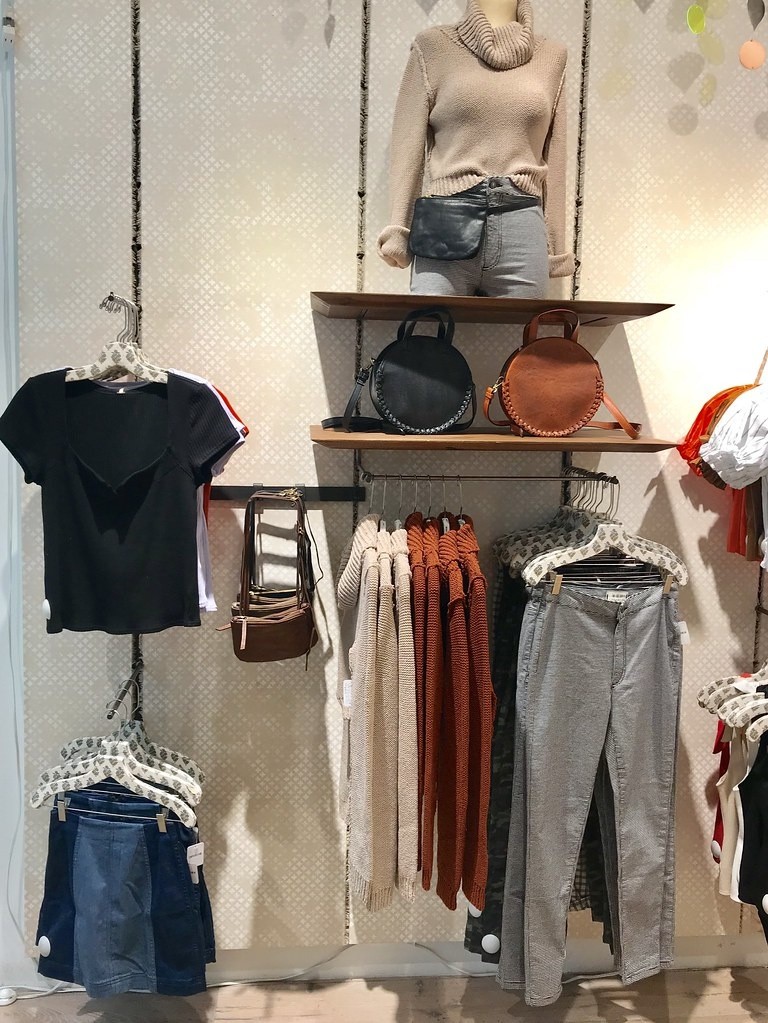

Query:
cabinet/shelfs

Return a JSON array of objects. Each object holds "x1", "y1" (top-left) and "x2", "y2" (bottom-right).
[{"x1": 308, "y1": 290, "x2": 684, "y2": 452}]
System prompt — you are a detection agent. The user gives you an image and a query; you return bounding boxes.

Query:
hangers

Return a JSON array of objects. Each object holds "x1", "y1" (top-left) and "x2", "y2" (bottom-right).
[
  {"x1": 29, "y1": 679, "x2": 206, "y2": 827},
  {"x1": 699, "y1": 649, "x2": 768, "y2": 742},
  {"x1": 66, "y1": 294, "x2": 169, "y2": 386},
  {"x1": 489, "y1": 466, "x2": 691, "y2": 596},
  {"x1": 367, "y1": 472, "x2": 464, "y2": 536}
]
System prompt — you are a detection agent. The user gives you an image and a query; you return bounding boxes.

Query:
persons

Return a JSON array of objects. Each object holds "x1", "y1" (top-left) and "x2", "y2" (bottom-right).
[{"x1": 376, "y1": 0, "x2": 575, "y2": 299}]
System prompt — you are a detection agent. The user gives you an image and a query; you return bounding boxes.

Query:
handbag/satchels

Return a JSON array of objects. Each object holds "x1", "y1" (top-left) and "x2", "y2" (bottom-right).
[
  {"x1": 409, "y1": 192, "x2": 487, "y2": 260},
  {"x1": 484, "y1": 307, "x2": 642, "y2": 438},
  {"x1": 215, "y1": 490, "x2": 319, "y2": 662},
  {"x1": 321, "y1": 308, "x2": 477, "y2": 434}
]
